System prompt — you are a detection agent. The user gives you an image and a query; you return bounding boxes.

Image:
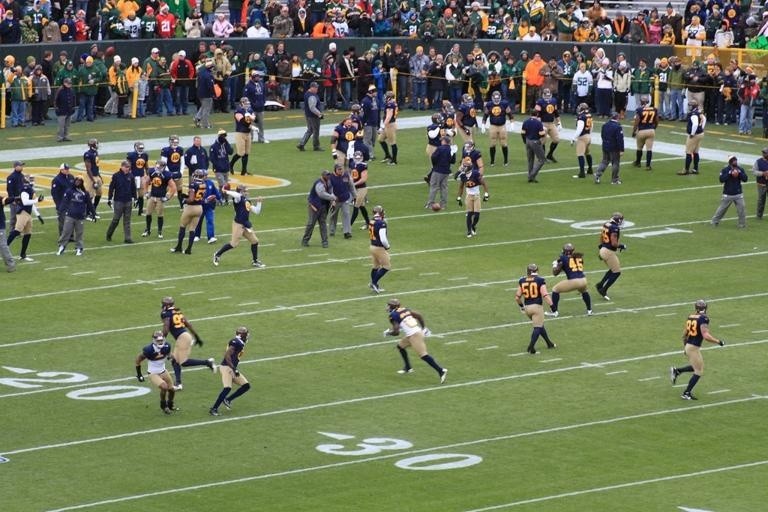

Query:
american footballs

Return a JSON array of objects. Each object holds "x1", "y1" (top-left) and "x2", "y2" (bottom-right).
[
  {"x1": 207, "y1": 195, "x2": 217, "y2": 202},
  {"x1": 106, "y1": 47, "x2": 114, "y2": 56},
  {"x1": 732, "y1": 169, "x2": 738, "y2": 177},
  {"x1": 224, "y1": 183, "x2": 230, "y2": 190},
  {"x1": 431, "y1": 203, "x2": 441, "y2": 211}
]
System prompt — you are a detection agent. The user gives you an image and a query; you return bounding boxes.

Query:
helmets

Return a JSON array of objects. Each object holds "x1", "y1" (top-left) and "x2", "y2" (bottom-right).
[
  {"x1": 611, "y1": 213, "x2": 624, "y2": 225},
  {"x1": 386, "y1": 299, "x2": 399, "y2": 313},
  {"x1": 639, "y1": 96, "x2": 650, "y2": 106},
  {"x1": 74, "y1": 175, "x2": 84, "y2": 186},
  {"x1": 87, "y1": 130, "x2": 248, "y2": 198},
  {"x1": 576, "y1": 103, "x2": 588, "y2": 115},
  {"x1": 323, "y1": 88, "x2": 396, "y2": 176},
  {"x1": 688, "y1": 100, "x2": 698, "y2": 111},
  {"x1": 562, "y1": 244, "x2": 574, "y2": 255},
  {"x1": 542, "y1": 87, "x2": 553, "y2": 100},
  {"x1": 527, "y1": 264, "x2": 538, "y2": 274},
  {"x1": 373, "y1": 204, "x2": 384, "y2": 220},
  {"x1": 238, "y1": 327, "x2": 250, "y2": 343},
  {"x1": 160, "y1": 295, "x2": 174, "y2": 307},
  {"x1": 23, "y1": 175, "x2": 35, "y2": 185},
  {"x1": 432, "y1": 91, "x2": 501, "y2": 173},
  {"x1": 152, "y1": 329, "x2": 165, "y2": 348},
  {"x1": 695, "y1": 301, "x2": 707, "y2": 314}
]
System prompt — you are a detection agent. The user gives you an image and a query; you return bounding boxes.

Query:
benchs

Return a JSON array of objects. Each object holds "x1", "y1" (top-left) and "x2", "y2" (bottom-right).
[
  {"x1": 569, "y1": 1, "x2": 686, "y2": 19},
  {"x1": 196, "y1": 0, "x2": 311, "y2": 24}
]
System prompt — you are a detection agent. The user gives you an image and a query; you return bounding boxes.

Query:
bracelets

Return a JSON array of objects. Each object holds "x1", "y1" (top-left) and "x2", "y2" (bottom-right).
[
  {"x1": 550, "y1": 304, "x2": 557, "y2": 312},
  {"x1": 136, "y1": 366, "x2": 142, "y2": 376},
  {"x1": 519, "y1": 304, "x2": 523, "y2": 307}
]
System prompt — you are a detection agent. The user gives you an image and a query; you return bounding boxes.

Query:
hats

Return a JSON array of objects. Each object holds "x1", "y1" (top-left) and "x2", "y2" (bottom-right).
[
  {"x1": 325, "y1": 43, "x2": 482, "y2": 68},
  {"x1": 4, "y1": 47, "x2": 186, "y2": 84},
  {"x1": 424, "y1": 0, "x2": 590, "y2": 33},
  {"x1": 13, "y1": 161, "x2": 26, "y2": 168},
  {"x1": 714, "y1": 8, "x2": 720, "y2": 14},
  {"x1": 6, "y1": 2, "x2": 225, "y2": 22},
  {"x1": 666, "y1": 2, "x2": 672, "y2": 8},
  {"x1": 762, "y1": 147, "x2": 768, "y2": 153},
  {"x1": 729, "y1": 155, "x2": 735, "y2": 163},
  {"x1": 59, "y1": 163, "x2": 69, "y2": 170},
  {"x1": 578, "y1": 53, "x2": 756, "y2": 79}
]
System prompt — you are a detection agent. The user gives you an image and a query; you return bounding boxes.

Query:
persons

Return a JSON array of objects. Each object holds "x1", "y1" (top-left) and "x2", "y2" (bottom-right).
[
  {"x1": 0, "y1": 0, "x2": 194, "y2": 127},
  {"x1": 520, "y1": 0, "x2": 768, "y2": 182},
  {"x1": 296, "y1": 82, "x2": 325, "y2": 152},
  {"x1": 544, "y1": 242, "x2": 593, "y2": 317},
  {"x1": 753, "y1": 148, "x2": 767, "y2": 219},
  {"x1": 712, "y1": 155, "x2": 749, "y2": 227},
  {"x1": 187, "y1": 0, "x2": 355, "y2": 126},
  {"x1": 346, "y1": 1, "x2": 521, "y2": 111},
  {"x1": 425, "y1": 91, "x2": 515, "y2": 235},
  {"x1": 595, "y1": 212, "x2": 627, "y2": 301},
  {"x1": 135, "y1": 330, "x2": 179, "y2": 414},
  {"x1": 361, "y1": 83, "x2": 398, "y2": 165},
  {"x1": 161, "y1": 297, "x2": 217, "y2": 392},
  {"x1": 55, "y1": 78, "x2": 75, "y2": 142},
  {"x1": 1, "y1": 139, "x2": 102, "y2": 272},
  {"x1": 368, "y1": 205, "x2": 391, "y2": 293},
  {"x1": 670, "y1": 299, "x2": 724, "y2": 400},
  {"x1": 301, "y1": 103, "x2": 371, "y2": 247},
  {"x1": 106, "y1": 127, "x2": 262, "y2": 267},
  {"x1": 209, "y1": 327, "x2": 250, "y2": 416},
  {"x1": 383, "y1": 298, "x2": 448, "y2": 384},
  {"x1": 515, "y1": 264, "x2": 558, "y2": 354}
]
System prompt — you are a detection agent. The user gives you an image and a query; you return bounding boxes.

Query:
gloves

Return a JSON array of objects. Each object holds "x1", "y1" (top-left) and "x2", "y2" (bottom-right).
[
  {"x1": 245, "y1": 112, "x2": 252, "y2": 118},
  {"x1": 38, "y1": 194, "x2": 43, "y2": 201},
  {"x1": 509, "y1": 123, "x2": 514, "y2": 131},
  {"x1": 456, "y1": 198, "x2": 462, "y2": 206},
  {"x1": 483, "y1": 195, "x2": 488, "y2": 202},
  {"x1": 384, "y1": 329, "x2": 390, "y2": 336},
  {"x1": 556, "y1": 122, "x2": 561, "y2": 130},
  {"x1": 719, "y1": 341, "x2": 724, "y2": 345},
  {"x1": 251, "y1": 125, "x2": 260, "y2": 132},
  {"x1": 422, "y1": 328, "x2": 430, "y2": 336},
  {"x1": 632, "y1": 130, "x2": 637, "y2": 136},
  {"x1": 621, "y1": 244, "x2": 627, "y2": 249},
  {"x1": 232, "y1": 367, "x2": 239, "y2": 376},
  {"x1": 139, "y1": 374, "x2": 144, "y2": 381}
]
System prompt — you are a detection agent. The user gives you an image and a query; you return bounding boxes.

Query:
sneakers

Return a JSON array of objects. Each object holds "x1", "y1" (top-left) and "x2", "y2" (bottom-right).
[
  {"x1": 682, "y1": 392, "x2": 697, "y2": 401},
  {"x1": 301, "y1": 223, "x2": 367, "y2": 248},
  {"x1": 19, "y1": 256, "x2": 34, "y2": 261},
  {"x1": 296, "y1": 144, "x2": 325, "y2": 152},
  {"x1": 141, "y1": 229, "x2": 217, "y2": 256},
  {"x1": 587, "y1": 310, "x2": 593, "y2": 315},
  {"x1": 528, "y1": 156, "x2": 623, "y2": 185},
  {"x1": 208, "y1": 357, "x2": 217, "y2": 375},
  {"x1": 56, "y1": 239, "x2": 84, "y2": 256},
  {"x1": 677, "y1": 168, "x2": 696, "y2": 175},
  {"x1": 13, "y1": 108, "x2": 189, "y2": 128},
  {"x1": 210, "y1": 397, "x2": 231, "y2": 416},
  {"x1": 368, "y1": 283, "x2": 385, "y2": 293},
  {"x1": 633, "y1": 161, "x2": 652, "y2": 170},
  {"x1": 397, "y1": 368, "x2": 414, "y2": 374},
  {"x1": 597, "y1": 284, "x2": 610, "y2": 301},
  {"x1": 671, "y1": 368, "x2": 680, "y2": 384},
  {"x1": 440, "y1": 368, "x2": 448, "y2": 383},
  {"x1": 251, "y1": 260, "x2": 265, "y2": 269},
  {"x1": 544, "y1": 311, "x2": 557, "y2": 317},
  {"x1": 214, "y1": 252, "x2": 221, "y2": 267},
  {"x1": 86, "y1": 214, "x2": 100, "y2": 221},
  {"x1": 193, "y1": 116, "x2": 214, "y2": 129},
  {"x1": 467, "y1": 225, "x2": 476, "y2": 237},
  {"x1": 739, "y1": 129, "x2": 751, "y2": 135},
  {"x1": 57, "y1": 136, "x2": 71, "y2": 142},
  {"x1": 160, "y1": 381, "x2": 183, "y2": 414}
]
